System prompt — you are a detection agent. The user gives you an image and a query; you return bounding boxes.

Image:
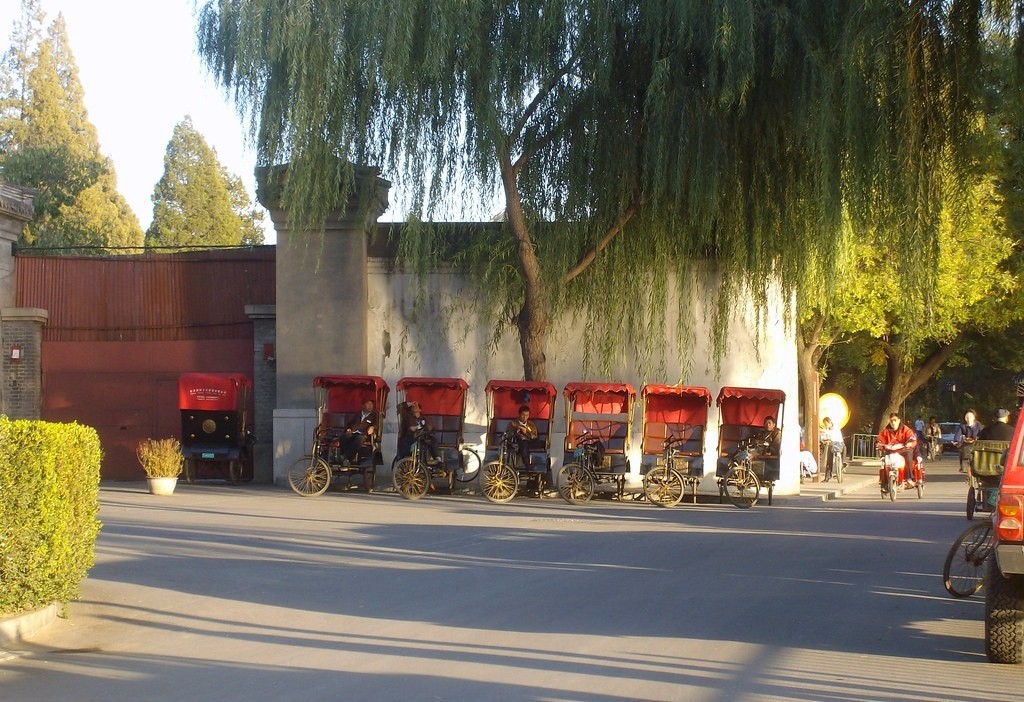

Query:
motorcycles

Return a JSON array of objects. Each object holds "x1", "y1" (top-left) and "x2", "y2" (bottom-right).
[
  {"x1": 958, "y1": 435, "x2": 980, "y2": 473},
  {"x1": 876, "y1": 441, "x2": 926, "y2": 501}
]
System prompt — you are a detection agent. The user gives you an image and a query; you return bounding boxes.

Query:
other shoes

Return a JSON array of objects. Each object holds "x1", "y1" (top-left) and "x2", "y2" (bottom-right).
[
  {"x1": 907, "y1": 478, "x2": 914, "y2": 485},
  {"x1": 344, "y1": 459, "x2": 350, "y2": 467}
]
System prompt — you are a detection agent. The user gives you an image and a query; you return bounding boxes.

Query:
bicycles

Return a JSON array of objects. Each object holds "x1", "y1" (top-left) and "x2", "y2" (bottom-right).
[
  {"x1": 943, "y1": 466, "x2": 1004, "y2": 599},
  {"x1": 455, "y1": 447, "x2": 481, "y2": 482},
  {"x1": 826, "y1": 439, "x2": 845, "y2": 484}
]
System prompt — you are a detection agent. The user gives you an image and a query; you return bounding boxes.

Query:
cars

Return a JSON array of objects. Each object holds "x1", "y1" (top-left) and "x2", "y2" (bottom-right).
[
  {"x1": 938, "y1": 421, "x2": 962, "y2": 450},
  {"x1": 983, "y1": 402, "x2": 1024, "y2": 666}
]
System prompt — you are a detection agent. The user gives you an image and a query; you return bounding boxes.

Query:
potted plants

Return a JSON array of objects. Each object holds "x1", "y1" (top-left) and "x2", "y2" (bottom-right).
[{"x1": 135, "y1": 435, "x2": 185, "y2": 495}]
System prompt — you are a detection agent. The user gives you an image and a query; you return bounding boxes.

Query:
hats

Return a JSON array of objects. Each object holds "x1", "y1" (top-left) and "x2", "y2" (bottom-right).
[
  {"x1": 995, "y1": 409, "x2": 1011, "y2": 417},
  {"x1": 361, "y1": 396, "x2": 375, "y2": 404}
]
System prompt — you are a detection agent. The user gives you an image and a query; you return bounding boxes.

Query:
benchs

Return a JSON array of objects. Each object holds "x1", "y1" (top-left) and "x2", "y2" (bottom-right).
[
  {"x1": 718, "y1": 424, "x2": 780, "y2": 459},
  {"x1": 640, "y1": 422, "x2": 705, "y2": 456},
  {"x1": 484, "y1": 418, "x2": 549, "y2": 452},
  {"x1": 323, "y1": 412, "x2": 377, "y2": 447},
  {"x1": 564, "y1": 420, "x2": 630, "y2": 453},
  {"x1": 421, "y1": 415, "x2": 460, "y2": 449}
]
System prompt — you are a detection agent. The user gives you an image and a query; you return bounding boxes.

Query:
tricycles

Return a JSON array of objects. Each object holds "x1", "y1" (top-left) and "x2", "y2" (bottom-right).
[
  {"x1": 393, "y1": 377, "x2": 470, "y2": 499},
  {"x1": 479, "y1": 379, "x2": 557, "y2": 505},
  {"x1": 177, "y1": 372, "x2": 258, "y2": 485},
  {"x1": 288, "y1": 374, "x2": 390, "y2": 497},
  {"x1": 640, "y1": 384, "x2": 713, "y2": 508},
  {"x1": 556, "y1": 381, "x2": 636, "y2": 506},
  {"x1": 716, "y1": 386, "x2": 788, "y2": 510}
]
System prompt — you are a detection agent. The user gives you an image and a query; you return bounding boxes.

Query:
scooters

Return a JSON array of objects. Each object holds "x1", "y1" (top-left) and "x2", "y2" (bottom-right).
[{"x1": 966, "y1": 440, "x2": 1012, "y2": 519}]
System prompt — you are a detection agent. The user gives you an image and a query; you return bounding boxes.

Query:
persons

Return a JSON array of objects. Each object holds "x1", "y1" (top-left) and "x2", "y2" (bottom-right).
[
  {"x1": 506, "y1": 406, "x2": 540, "y2": 470},
  {"x1": 819, "y1": 417, "x2": 848, "y2": 482},
  {"x1": 970, "y1": 409, "x2": 1015, "y2": 484},
  {"x1": 952, "y1": 409, "x2": 983, "y2": 473},
  {"x1": 339, "y1": 398, "x2": 378, "y2": 468},
  {"x1": 875, "y1": 413, "x2": 917, "y2": 492},
  {"x1": 396, "y1": 400, "x2": 447, "y2": 468},
  {"x1": 905, "y1": 416, "x2": 941, "y2": 460},
  {"x1": 726, "y1": 416, "x2": 781, "y2": 470}
]
